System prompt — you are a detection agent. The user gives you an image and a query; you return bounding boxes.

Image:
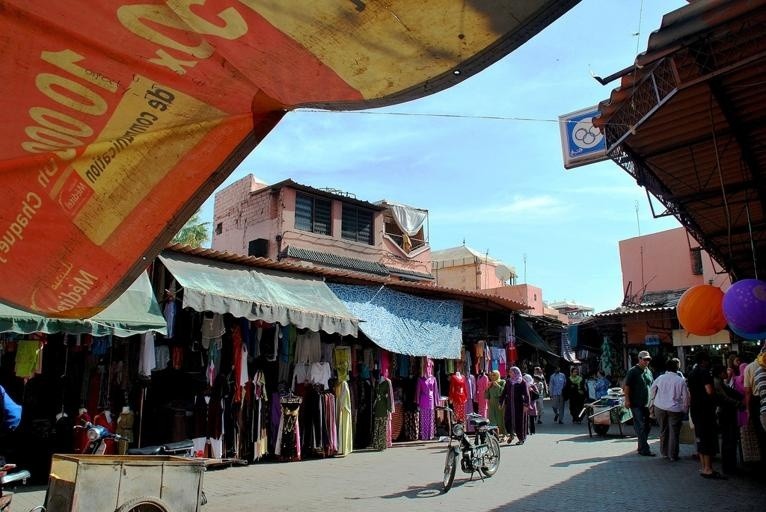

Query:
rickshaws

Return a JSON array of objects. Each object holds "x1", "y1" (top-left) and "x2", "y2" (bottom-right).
[
  {"x1": 41, "y1": 449, "x2": 248, "y2": 512},
  {"x1": 578, "y1": 387, "x2": 631, "y2": 438}
]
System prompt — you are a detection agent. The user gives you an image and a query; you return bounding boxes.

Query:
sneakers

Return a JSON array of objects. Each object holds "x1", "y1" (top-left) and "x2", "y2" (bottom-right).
[
  {"x1": 537, "y1": 413, "x2": 581, "y2": 424},
  {"x1": 638, "y1": 451, "x2": 680, "y2": 461}
]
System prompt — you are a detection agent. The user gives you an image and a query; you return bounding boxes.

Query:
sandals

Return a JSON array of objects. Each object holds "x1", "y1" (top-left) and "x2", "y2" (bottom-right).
[
  {"x1": 507, "y1": 436, "x2": 514, "y2": 444},
  {"x1": 516, "y1": 441, "x2": 524, "y2": 445}
]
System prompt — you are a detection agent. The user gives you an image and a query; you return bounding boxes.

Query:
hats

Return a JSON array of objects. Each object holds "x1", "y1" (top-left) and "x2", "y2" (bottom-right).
[{"x1": 637, "y1": 350, "x2": 652, "y2": 361}]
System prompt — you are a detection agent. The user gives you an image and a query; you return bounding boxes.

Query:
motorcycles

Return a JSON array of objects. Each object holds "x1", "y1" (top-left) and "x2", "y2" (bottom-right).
[
  {"x1": 72, "y1": 413, "x2": 209, "y2": 507},
  {"x1": 433, "y1": 404, "x2": 501, "y2": 492}
]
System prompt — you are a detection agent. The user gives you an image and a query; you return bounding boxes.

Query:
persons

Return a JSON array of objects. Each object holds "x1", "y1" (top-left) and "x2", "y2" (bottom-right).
[
  {"x1": 623, "y1": 345, "x2": 766, "y2": 479},
  {"x1": 563, "y1": 367, "x2": 585, "y2": 422},
  {"x1": 448, "y1": 369, "x2": 489, "y2": 422},
  {"x1": 54, "y1": 405, "x2": 134, "y2": 456},
  {"x1": 487, "y1": 365, "x2": 549, "y2": 446},
  {"x1": 548, "y1": 366, "x2": 567, "y2": 424},
  {"x1": 373, "y1": 375, "x2": 395, "y2": 451},
  {"x1": 415, "y1": 375, "x2": 440, "y2": 440},
  {"x1": 593, "y1": 370, "x2": 612, "y2": 400}
]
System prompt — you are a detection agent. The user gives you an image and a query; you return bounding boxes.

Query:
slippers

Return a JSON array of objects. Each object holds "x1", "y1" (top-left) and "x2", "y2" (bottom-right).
[{"x1": 700, "y1": 471, "x2": 720, "y2": 480}]
